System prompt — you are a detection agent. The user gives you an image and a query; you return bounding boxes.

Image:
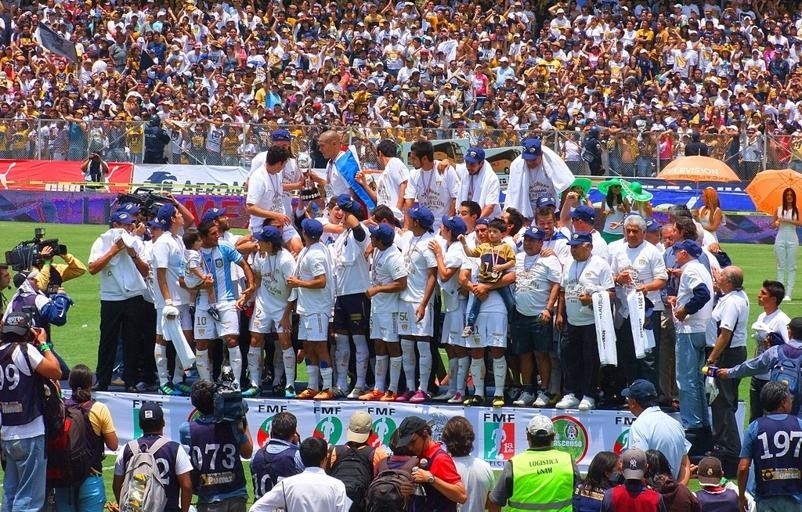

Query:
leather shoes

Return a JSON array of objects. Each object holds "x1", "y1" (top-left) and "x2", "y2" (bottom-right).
[
  {"x1": 599, "y1": 394, "x2": 624, "y2": 406},
  {"x1": 684, "y1": 424, "x2": 711, "y2": 433},
  {"x1": 157, "y1": 382, "x2": 193, "y2": 396},
  {"x1": 127, "y1": 381, "x2": 157, "y2": 392},
  {"x1": 705, "y1": 446, "x2": 721, "y2": 456},
  {"x1": 110, "y1": 378, "x2": 125, "y2": 385},
  {"x1": 91, "y1": 382, "x2": 108, "y2": 390},
  {"x1": 272, "y1": 384, "x2": 351, "y2": 399},
  {"x1": 432, "y1": 389, "x2": 505, "y2": 407},
  {"x1": 506, "y1": 386, "x2": 597, "y2": 410},
  {"x1": 347, "y1": 386, "x2": 431, "y2": 403}
]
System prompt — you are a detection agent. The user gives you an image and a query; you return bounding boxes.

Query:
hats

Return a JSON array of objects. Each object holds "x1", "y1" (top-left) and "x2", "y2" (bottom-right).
[
  {"x1": 622, "y1": 450, "x2": 646, "y2": 480},
  {"x1": 346, "y1": 411, "x2": 373, "y2": 443},
  {"x1": 3, "y1": 312, "x2": 31, "y2": 336},
  {"x1": 697, "y1": 458, "x2": 722, "y2": 486},
  {"x1": 621, "y1": 379, "x2": 658, "y2": 398},
  {"x1": 787, "y1": 317, "x2": 802, "y2": 332},
  {"x1": 138, "y1": 402, "x2": 162, "y2": 427},
  {"x1": 397, "y1": 417, "x2": 427, "y2": 448},
  {"x1": 528, "y1": 416, "x2": 554, "y2": 435},
  {"x1": 337, "y1": 194, "x2": 361, "y2": 211},
  {"x1": 462, "y1": 147, "x2": 485, "y2": 163},
  {"x1": 409, "y1": 208, "x2": 491, "y2": 235},
  {"x1": 523, "y1": 197, "x2": 597, "y2": 246},
  {"x1": 13, "y1": 272, "x2": 37, "y2": 288},
  {"x1": 109, "y1": 203, "x2": 174, "y2": 231},
  {"x1": 560, "y1": 179, "x2": 653, "y2": 202},
  {"x1": 523, "y1": 138, "x2": 540, "y2": 162},
  {"x1": 369, "y1": 224, "x2": 394, "y2": 243},
  {"x1": 676, "y1": 240, "x2": 702, "y2": 257},
  {"x1": 301, "y1": 218, "x2": 324, "y2": 239},
  {"x1": 254, "y1": 226, "x2": 282, "y2": 243},
  {"x1": 197, "y1": 207, "x2": 224, "y2": 232}
]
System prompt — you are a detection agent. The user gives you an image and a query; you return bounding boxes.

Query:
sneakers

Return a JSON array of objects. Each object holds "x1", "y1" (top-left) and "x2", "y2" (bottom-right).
[
  {"x1": 241, "y1": 387, "x2": 263, "y2": 397},
  {"x1": 208, "y1": 307, "x2": 220, "y2": 322},
  {"x1": 461, "y1": 325, "x2": 474, "y2": 338},
  {"x1": 189, "y1": 306, "x2": 195, "y2": 315},
  {"x1": 783, "y1": 295, "x2": 792, "y2": 301},
  {"x1": 262, "y1": 376, "x2": 274, "y2": 385}
]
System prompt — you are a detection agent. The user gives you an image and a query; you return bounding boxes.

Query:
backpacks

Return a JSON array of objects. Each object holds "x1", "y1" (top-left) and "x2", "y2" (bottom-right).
[
  {"x1": 64, "y1": 407, "x2": 105, "y2": 466},
  {"x1": 365, "y1": 456, "x2": 419, "y2": 512},
  {"x1": 331, "y1": 446, "x2": 376, "y2": 512},
  {"x1": 118, "y1": 437, "x2": 173, "y2": 512}
]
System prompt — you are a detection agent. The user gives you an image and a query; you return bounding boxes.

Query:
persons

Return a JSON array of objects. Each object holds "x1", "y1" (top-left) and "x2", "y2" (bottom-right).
[
  {"x1": 2, "y1": 0, "x2": 801, "y2": 190},
  {"x1": 1, "y1": 132, "x2": 801, "y2": 511}
]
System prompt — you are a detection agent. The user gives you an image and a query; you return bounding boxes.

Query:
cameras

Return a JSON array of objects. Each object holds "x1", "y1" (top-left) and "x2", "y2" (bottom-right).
[{"x1": 21, "y1": 305, "x2": 36, "y2": 329}]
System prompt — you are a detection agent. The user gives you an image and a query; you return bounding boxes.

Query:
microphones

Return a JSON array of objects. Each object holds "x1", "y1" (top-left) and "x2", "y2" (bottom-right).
[
  {"x1": 701, "y1": 365, "x2": 718, "y2": 378},
  {"x1": 7, "y1": 285, "x2": 11, "y2": 289}
]
